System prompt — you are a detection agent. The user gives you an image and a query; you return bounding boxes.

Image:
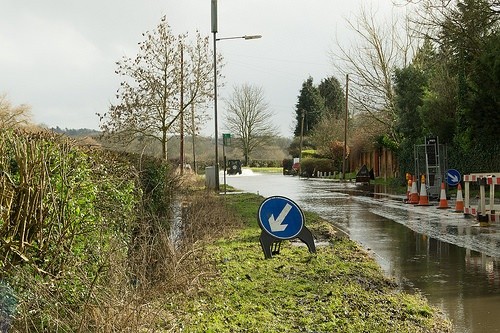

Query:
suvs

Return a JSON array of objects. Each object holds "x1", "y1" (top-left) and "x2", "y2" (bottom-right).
[{"x1": 228, "y1": 159, "x2": 243, "y2": 175}]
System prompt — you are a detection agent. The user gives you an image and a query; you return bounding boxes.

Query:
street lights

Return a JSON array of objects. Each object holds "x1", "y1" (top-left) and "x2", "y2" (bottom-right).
[
  {"x1": 189, "y1": 80, "x2": 213, "y2": 174},
  {"x1": 213, "y1": 35, "x2": 263, "y2": 192},
  {"x1": 299, "y1": 108, "x2": 321, "y2": 177}
]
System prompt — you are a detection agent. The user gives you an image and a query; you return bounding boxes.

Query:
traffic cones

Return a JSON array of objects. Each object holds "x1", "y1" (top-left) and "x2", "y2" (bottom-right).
[
  {"x1": 414, "y1": 175, "x2": 435, "y2": 206},
  {"x1": 404, "y1": 175, "x2": 419, "y2": 204},
  {"x1": 447, "y1": 183, "x2": 464, "y2": 213},
  {"x1": 403, "y1": 174, "x2": 412, "y2": 202},
  {"x1": 436, "y1": 181, "x2": 452, "y2": 209}
]
